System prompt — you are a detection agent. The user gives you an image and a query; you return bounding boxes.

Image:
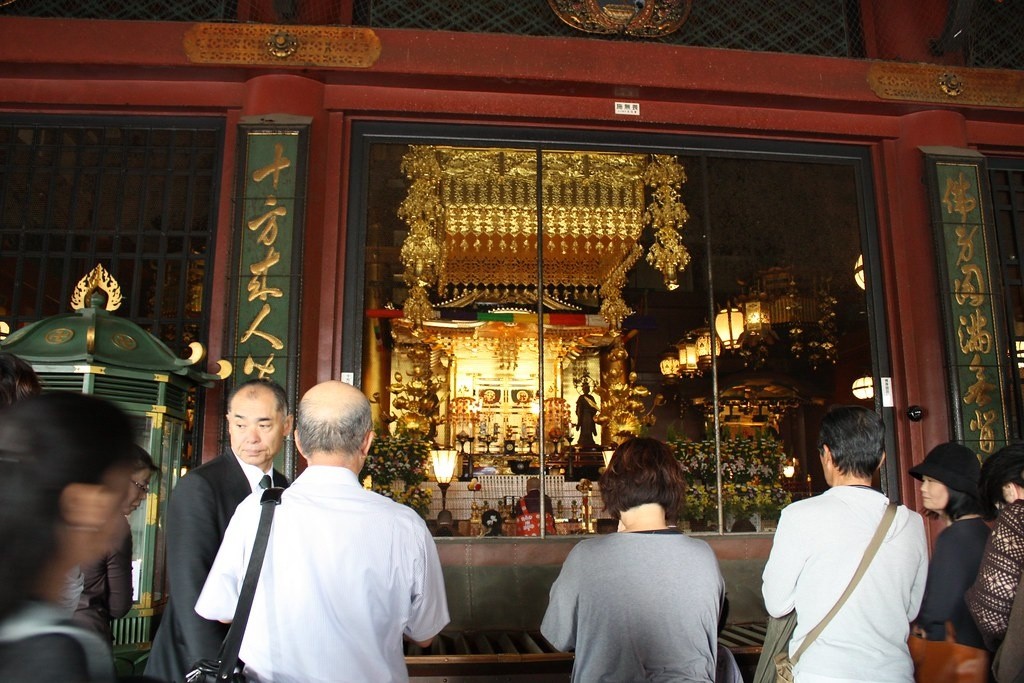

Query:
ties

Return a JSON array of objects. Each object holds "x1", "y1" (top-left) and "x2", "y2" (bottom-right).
[{"x1": 259, "y1": 475, "x2": 272, "y2": 490}]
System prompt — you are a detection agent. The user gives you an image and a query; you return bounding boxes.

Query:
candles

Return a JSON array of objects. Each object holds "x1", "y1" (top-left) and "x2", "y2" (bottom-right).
[{"x1": 468, "y1": 423, "x2": 473, "y2": 438}]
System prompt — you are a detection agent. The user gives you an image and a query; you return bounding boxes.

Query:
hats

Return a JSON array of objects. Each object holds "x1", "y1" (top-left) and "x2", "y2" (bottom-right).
[{"x1": 907, "y1": 442, "x2": 981, "y2": 497}]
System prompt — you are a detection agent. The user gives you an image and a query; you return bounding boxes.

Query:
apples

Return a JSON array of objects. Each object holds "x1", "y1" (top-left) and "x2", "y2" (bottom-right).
[{"x1": 468, "y1": 478, "x2": 482, "y2": 490}]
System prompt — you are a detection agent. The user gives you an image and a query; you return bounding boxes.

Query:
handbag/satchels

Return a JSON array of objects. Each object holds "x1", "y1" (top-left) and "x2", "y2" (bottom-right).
[
  {"x1": 905, "y1": 618, "x2": 990, "y2": 683},
  {"x1": 185, "y1": 657, "x2": 247, "y2": 683},
  {"x1": 715, "y1": 644, "x2": 745, "y2": 683},
  {"x1": 773, "y1": 652, "x2": 797, "y2": 683},
  {"x1": 516, "y1": 499, "x2": 557, "y2": 536}
]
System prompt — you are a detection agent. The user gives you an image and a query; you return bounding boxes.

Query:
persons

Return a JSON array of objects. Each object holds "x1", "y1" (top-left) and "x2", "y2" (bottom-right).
[
  {"x1": 908, "y1": 441, "x2": 1024, "y2": 683},
  {"x1": 540, "y1": 438, "x2": 743, "y2": 683},
  {"x1": 761, "y1": 405, "x2": 929, "y2": 683},
  {"x1": 576, "y1": 384, "x2": 599, "y2": 450},
  {"x1": 514, "y1": 477, "x2": 556, "y2": 528},
  {"x1": 1, "y1": 352, "x2": 450, "y2": 683}
]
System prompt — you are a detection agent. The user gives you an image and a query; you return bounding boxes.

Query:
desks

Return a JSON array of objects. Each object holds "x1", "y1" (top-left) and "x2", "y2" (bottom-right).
[
  {"x1": 468, "y1": 519, "x2": 583, "y2": 536},
  {"x1": 457, "y1": 453, "x2": 534, "y2": 462}
]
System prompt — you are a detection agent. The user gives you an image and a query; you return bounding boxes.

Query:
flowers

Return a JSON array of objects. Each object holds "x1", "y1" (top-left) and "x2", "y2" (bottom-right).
[
  {"x1": 666, "y1": 435, "x2": 795, "y2": 521},
  {"x1": 358, "y1": 424, "x2": 432, "y2": 523}
]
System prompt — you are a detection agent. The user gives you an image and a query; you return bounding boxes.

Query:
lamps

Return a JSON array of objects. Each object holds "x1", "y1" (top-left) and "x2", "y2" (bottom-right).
[
  {"x1": 396, "y1": 145, "x2": 690, "y2": 337},
  {"x1": 431, "y1": 449, "x2": 457, "y2": 512}
]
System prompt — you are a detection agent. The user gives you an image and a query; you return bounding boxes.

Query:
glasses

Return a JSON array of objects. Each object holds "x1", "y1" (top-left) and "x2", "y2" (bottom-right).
[{"x1": 131, "y1": 479, "x2": 150, "y2": 494}]
